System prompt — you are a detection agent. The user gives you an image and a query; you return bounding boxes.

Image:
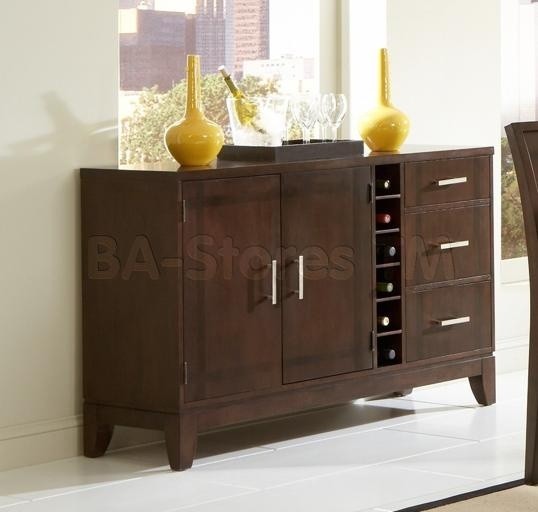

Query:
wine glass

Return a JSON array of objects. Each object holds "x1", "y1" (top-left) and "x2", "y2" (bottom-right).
[
  {"x1": 274, "y1": 98, "x2": 293, "y2": 145},
  {"x1": 291, "y1": 97, "x2": 306, "y2": 145},
  {"x1": 316, "y1": 94, "x2": 328, "y2": 143},
  {"x1": 296, "y1": 95, "x2": 316, "y2": 144},
  {"x1": 323, "y1": 94, "x2": 347, "y2": 142}
]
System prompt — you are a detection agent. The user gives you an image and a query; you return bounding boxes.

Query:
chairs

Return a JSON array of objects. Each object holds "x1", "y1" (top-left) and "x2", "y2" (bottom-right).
[{"x1": 503, "y1": 120, "x2": 538, "y2": 488}]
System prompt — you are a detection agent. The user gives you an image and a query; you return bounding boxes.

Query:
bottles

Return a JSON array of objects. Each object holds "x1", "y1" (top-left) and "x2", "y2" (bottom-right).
[{"x1": 218, "y1": 65, "x2": 274, "y2": 139}]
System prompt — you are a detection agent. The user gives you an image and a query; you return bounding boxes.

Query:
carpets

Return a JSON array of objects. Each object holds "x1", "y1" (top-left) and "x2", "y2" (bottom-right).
[{"x1": 393, "y1": 479, "x2": 538, "y2": 512}]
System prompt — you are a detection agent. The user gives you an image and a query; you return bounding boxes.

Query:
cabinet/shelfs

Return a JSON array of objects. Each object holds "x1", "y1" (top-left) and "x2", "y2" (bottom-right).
[
  {"x1": 81, "y1": 167, "x2": 373, "y2": 402},
  {"x1": 374, "y1": 157, "x2": 490, "y2": 368}
]
